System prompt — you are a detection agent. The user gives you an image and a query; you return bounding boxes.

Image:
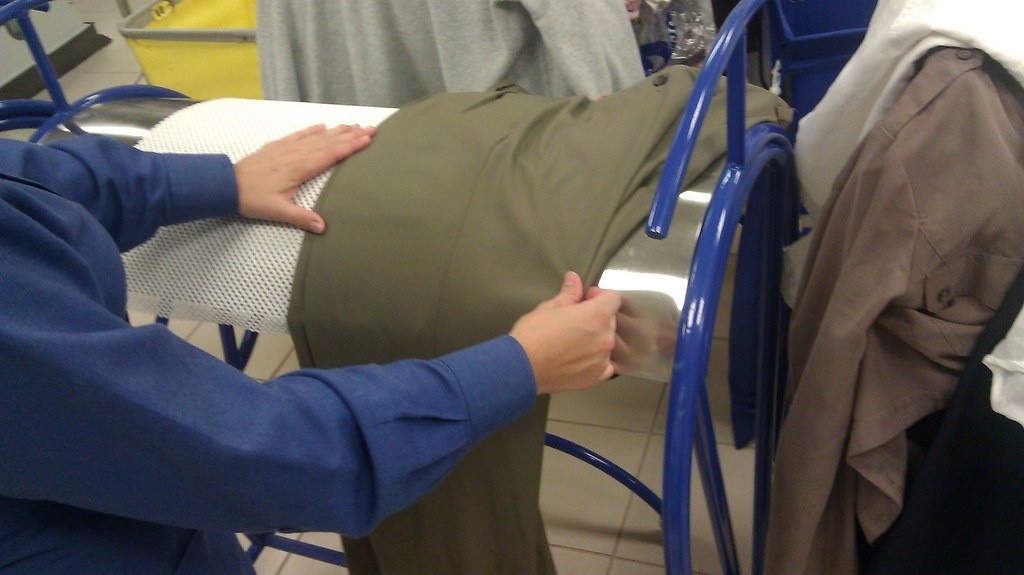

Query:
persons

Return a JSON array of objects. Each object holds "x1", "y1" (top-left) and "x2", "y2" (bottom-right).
[{"x1": 0, "y1": 123, "x2": 622, "y2": 575}]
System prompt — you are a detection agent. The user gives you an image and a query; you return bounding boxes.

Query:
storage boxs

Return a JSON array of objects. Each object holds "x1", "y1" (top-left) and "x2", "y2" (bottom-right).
[{"x1": 117, "y1": 0, "x2": 263, "y2": 101}]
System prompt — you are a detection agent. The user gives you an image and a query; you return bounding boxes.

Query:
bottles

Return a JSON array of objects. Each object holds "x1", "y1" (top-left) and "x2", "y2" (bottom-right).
[{"x1": 660, "y1": 0, "x2": 706, "y2": 66}]
